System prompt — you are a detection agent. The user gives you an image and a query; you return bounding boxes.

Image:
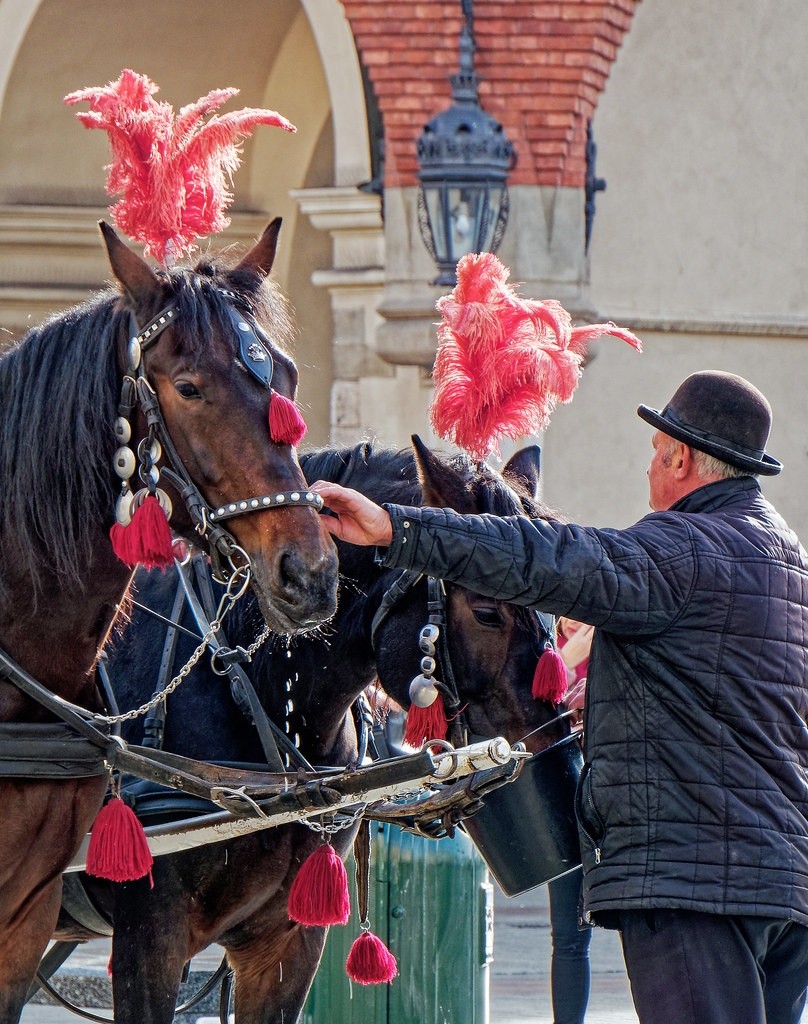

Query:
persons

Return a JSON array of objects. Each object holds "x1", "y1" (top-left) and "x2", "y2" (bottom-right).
[{"x1": 308, "y1": 370, "x2": 808, "y2": 1024}]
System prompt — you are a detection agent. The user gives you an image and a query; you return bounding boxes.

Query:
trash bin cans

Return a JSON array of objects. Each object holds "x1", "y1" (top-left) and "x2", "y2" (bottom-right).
[{"x1": 299, "y1": 792, "x2": 495, "y2": 1023}]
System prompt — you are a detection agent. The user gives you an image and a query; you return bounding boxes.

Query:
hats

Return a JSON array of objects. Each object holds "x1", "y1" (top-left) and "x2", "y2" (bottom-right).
[{"x1": 637, "y1": 369, "x2": 784, "y2": 476}]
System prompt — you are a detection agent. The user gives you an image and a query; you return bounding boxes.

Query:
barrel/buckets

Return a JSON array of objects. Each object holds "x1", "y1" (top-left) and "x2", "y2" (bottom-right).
[{"x1": 459, "y1": 707, "x2": 587, "y2": 898}]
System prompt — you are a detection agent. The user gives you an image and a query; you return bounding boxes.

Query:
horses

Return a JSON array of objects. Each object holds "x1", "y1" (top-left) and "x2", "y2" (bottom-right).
[{"x1": 0, "y1": 217, "x2": 578, "y2": 1024}]
